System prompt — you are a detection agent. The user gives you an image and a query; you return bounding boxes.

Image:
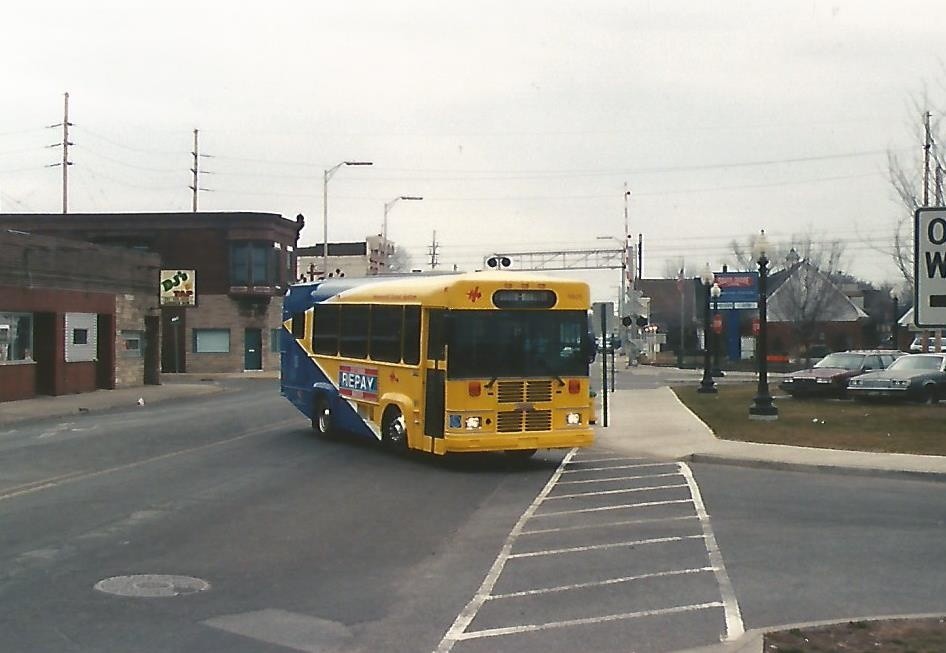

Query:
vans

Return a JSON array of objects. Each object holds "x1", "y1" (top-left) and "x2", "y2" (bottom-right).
[{"x1": 910, "y1": 337, "x2": 946, "y2": 353}]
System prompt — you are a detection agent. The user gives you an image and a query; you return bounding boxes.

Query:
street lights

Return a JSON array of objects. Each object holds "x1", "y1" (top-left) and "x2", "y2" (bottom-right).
[
  {"x1": 382, "y1": 198, "x2": 422, "y2": 273},
  {"x1": 749, "y1": 229, "x2": 779, "y2": 420},
  {"x1": 696, "y1": 261, "x2": 720, "y2": 393},
  {"x1": 323, "y1": 161, "x2": 375, "y2": 279},
  {"x1": 710, "y1": 282, "x2": 726, "y2": 377}
]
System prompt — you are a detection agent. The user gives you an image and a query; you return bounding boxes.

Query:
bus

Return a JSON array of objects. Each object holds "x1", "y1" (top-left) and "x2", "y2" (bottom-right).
[{"x1": 280, "y1": 268, "x2": 598, "y2": 461}]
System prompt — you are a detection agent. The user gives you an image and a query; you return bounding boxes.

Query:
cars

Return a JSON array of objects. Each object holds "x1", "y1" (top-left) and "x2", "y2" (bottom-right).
[
  {"x1": 778, "y1": 349, "x2": 911, "y2": 400},
  {"x1": 594, "y1": 333, "x2": 619, "y2": 354},
  {"x1": 847, "y1": 352, "x2": 946, "y2": 405}
]
